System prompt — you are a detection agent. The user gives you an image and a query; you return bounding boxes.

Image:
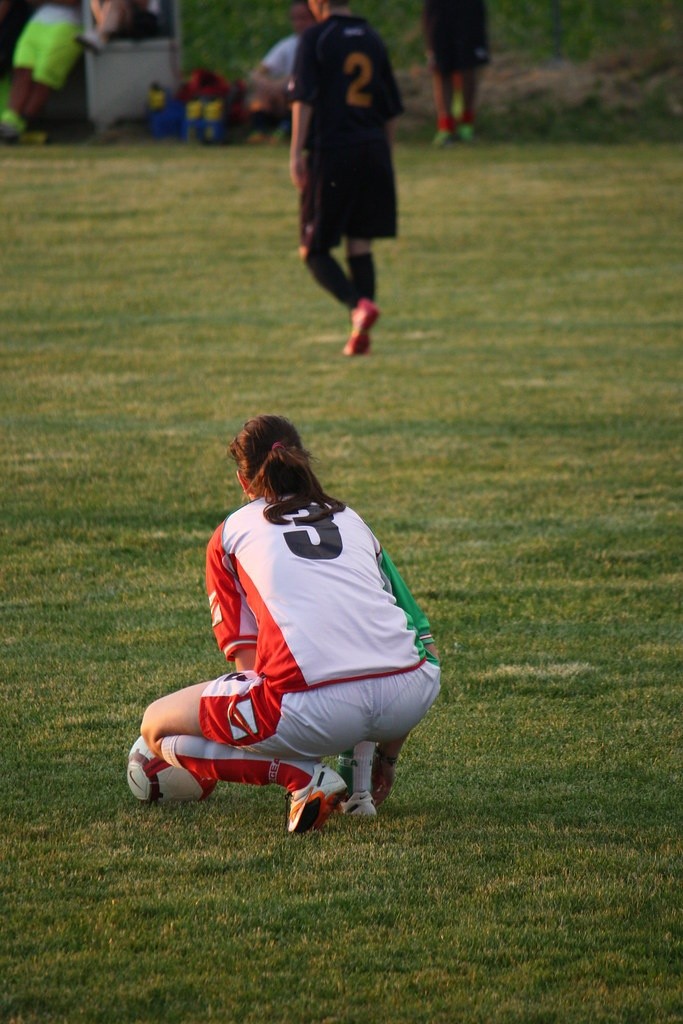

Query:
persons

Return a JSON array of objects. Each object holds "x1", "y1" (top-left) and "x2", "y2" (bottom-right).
[
  {"x1": 140, "y1": 415, "x2": 441, "y2": 834},
  {"x1": 290, "y1": 0, "x2": 406, "y2": 357},
  {"x1": 0, "y1": 0, "x2": 84, "y2": 140},
  {"x1": 422, "y1": 0, "x2": 490, "y2": 149},
  {"x1": 76, "y1": 0, "x2": 165, "y2": 54},
  {"x1": 248, "y1": 0, "x2": 318, "y2": 143}
]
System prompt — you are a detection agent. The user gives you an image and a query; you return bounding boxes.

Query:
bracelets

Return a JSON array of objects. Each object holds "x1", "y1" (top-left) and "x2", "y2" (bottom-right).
[{"x1": 377, "y1": 755, "x2": 398, "y2": 765}]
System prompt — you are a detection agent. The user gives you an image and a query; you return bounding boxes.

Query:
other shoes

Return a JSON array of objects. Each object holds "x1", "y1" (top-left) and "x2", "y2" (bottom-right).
[
  {"x1": 457, "y1": 124, "x2": 476, "y2": 142},
  {"x1": 432, "y1": 130, "x2": 456, "y2": 146}
]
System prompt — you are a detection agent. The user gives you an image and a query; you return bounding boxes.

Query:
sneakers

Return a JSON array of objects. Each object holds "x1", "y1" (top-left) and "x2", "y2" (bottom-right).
[
  {"x1": 285, "y1": 761, "x2": 348, "y2": 834},
  {"x1": 342, "y1": 298, "x2": 383, "y2": 357},
  {"x1": 340, "y1": 791, "x2": 379, "y2": 818}
]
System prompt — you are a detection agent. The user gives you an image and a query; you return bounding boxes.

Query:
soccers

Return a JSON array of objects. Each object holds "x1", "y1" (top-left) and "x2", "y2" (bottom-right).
[{"x1": 127, "y1": 735, "x2": 217, "y2": 800}]
